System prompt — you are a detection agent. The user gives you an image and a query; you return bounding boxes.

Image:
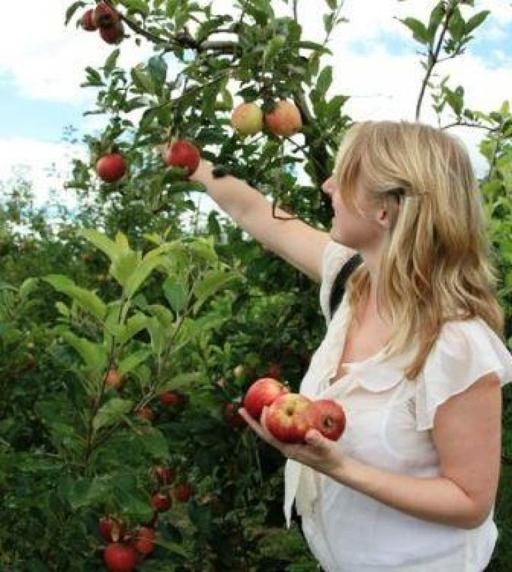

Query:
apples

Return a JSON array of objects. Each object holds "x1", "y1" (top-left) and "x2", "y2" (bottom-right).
[
  {"x1": 81, "y1": 0, "x2": 126, "y2": 43},
  {"x1": 303, "y1": 399, "x2": 344, "y2": 442},
  {"x1": 95, "y1": 154, "x2": 127, "y2": 184},
  {"x1": 265, "y1": 391, "x2": 311, "y2": 444},
  {"x1": 232, "y1": 100, "x2": 302, "y2": 135},
  {"x1": 165, "y1": 140, "x2": 202, "y2": 175},
  {"x1": 244, "y1": 378, "x2": 289, "y2": 423},
  {"x1": 95, "y1": 362, "x2": 279, "y2": 572}
]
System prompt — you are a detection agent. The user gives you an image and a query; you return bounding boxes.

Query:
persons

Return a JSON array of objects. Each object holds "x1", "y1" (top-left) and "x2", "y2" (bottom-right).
[{"x1": 190, "y1": 121, "x2": 512, "y2": 572}]
